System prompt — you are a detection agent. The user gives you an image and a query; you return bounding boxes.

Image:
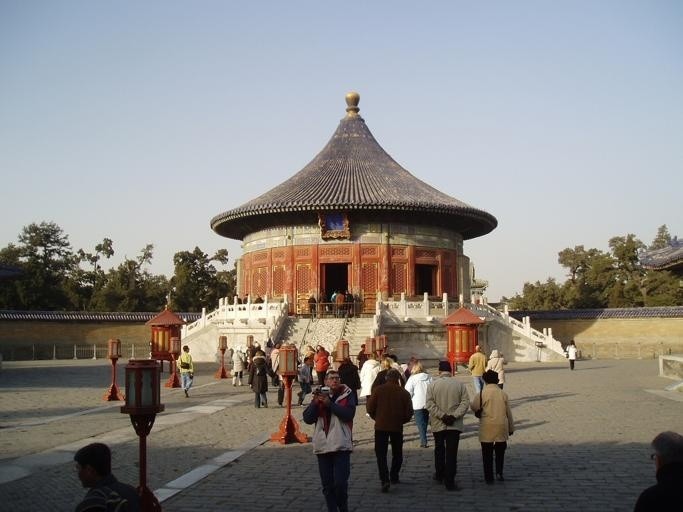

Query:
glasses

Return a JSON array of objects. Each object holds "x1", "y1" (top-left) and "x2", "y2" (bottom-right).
[{"x1": 328, "y1": 376, "x2": 341, "y2": 381}]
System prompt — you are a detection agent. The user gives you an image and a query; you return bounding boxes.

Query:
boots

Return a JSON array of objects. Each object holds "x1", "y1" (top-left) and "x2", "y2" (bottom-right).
[
  {"x1": 232, "y1": 377, "x2": 237, "y2": 386},
  {"x1": 238, "y1": 378, "x2": 243, "y2": 386}
]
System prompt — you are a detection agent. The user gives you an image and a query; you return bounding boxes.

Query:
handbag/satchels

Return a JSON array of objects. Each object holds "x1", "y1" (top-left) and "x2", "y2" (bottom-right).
[{"x1": 181, "y1": 362, "x2": 189, "y2": 369}]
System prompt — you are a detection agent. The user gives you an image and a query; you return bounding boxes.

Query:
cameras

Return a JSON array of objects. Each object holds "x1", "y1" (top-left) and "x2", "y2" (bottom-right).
[{"x1": 315, "y1": 385, "x2": 330, "y2": 394}]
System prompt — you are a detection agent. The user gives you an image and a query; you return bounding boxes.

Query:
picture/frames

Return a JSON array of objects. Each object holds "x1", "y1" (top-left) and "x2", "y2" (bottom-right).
[{"x1": 318, "y1": 211, "x2": 352, "y2": 241}]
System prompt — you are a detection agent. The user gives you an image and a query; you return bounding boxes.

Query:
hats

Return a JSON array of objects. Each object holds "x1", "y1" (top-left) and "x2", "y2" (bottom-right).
[
  {"x1": 483, "y1": 369, "x2": 500, "y2": 384},
  {"x1": 438, "y1": 361, "x2": 452, "y2": 372}
]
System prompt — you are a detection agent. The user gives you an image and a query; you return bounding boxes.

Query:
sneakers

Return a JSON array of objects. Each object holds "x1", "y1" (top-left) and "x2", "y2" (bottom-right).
[
  {"x1": 420, "y1": 442, "x2": 428, "y2": 448},
  {"x1": 381, "y1": 480, "x2": 389, "y2": 492},
  {"x1": 390, "y1": 475, "x2": 399, "y2": 483},
  {"x1": 433, "y1": 473, "x2": 443, "y2": 484},
  {"x1": 486, "y1": 477, "x2": 494, "y2": 484},
  {"x1": 297, "y1": 392, "x2": 303, "y2": 406},
  {"x1": 183, "y1": 388, "x2": 188, "y2": 398},
  {"x1": 445, "y1": 479, "x2": 463, "y2": 491},
  {"x1": 496, "y1": 474, "x2": 504, "y2": 481},
  {"x1": 255, "y1": 403, "x2": 268, "y2": 409}
]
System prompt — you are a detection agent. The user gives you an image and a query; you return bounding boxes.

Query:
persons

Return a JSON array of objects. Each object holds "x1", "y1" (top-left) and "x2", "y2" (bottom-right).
[
  {"x1": 176, "y1": 346, "x2": 193, "y2": 398},
  {"x1": 470, "y1": 370, "x2": 514, "y2": 486},
  {"x1": 632, "y1": 432, "x2": 681, "y2": 512},
  {"x1": 425, "y1": 360, "x2": 469, "y2": 491},
  {"x1": 365, "y1": 368, "x2": 414, "y2": 493},
  {"x1": 233, "y1": 288, "x2": 508, "y2": 449},
  {"x1": 565, "y1": 339, "x2": 577, "y2": 371},
  {"x1": 303, "y1": 369, "x2": 357, "y2": 512},
  {"x1": 73, "y1": 443, "x2": 145, "y2": 512}
]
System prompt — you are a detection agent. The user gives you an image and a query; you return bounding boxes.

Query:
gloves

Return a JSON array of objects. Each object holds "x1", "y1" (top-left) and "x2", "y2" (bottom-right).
[
  {"x1": 447, "y1": 415, "x2": 455, "y2": 426},
  {"x1": 443, "y1": 415, "x2": 450, "y2": 424}
]
